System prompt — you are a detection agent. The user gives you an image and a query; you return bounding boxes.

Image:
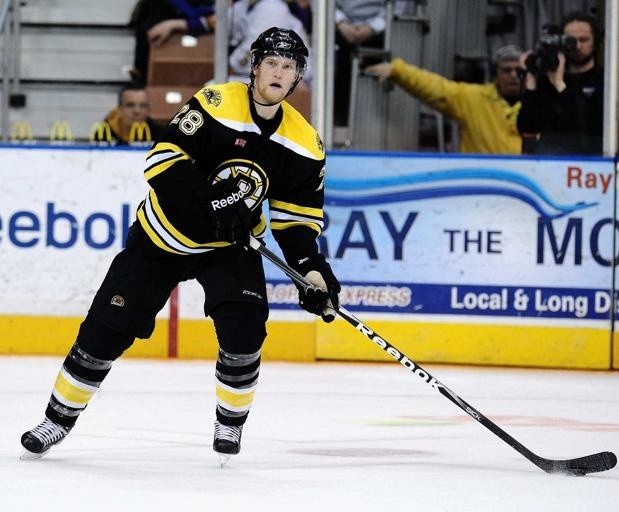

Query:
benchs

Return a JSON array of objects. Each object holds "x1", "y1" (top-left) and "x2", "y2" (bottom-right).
[{"x1": 0, "y1": 1, "x2": 143, "y2": 142}]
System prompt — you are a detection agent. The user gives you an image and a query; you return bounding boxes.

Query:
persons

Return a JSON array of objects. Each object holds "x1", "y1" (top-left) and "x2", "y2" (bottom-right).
[
  {"x1": 125, "y1": 0, "x2": 217, "y2": 83},
  {"x1": 19, "y1": 28, "x2": 344, "y2": 457},
  {"x1": 227, "y1": 1, "x2": 315, "y2": 86},
  {"x1": 364, "y1": 44, "x2": 525, "y2": 155},
  {"x1": 514, "y1": 6, "x2": 605, "y2": 156},
  {"x1": 334, "y1": 0, "x2": 419, "y2": 127},
  {"x1": 93, "y1": 84, "x2": 161, "y2": 142}
]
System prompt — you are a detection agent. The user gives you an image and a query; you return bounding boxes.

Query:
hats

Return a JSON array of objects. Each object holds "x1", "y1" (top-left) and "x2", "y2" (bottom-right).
[{"x1": 492, "y1": 45, "x2": 522, "y2": 66}]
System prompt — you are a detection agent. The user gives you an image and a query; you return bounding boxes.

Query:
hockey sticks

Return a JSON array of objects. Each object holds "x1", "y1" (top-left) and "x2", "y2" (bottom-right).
[{"x1": 247, "y1": 235, "x2": 617, "y2": 473}]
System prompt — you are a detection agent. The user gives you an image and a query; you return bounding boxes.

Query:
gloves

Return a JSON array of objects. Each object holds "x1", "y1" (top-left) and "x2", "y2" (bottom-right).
[
  {"x1": 364, "y1": 62, "x2": 391, "y2": 85},
  {"x1": 291, "y1": 254, "x2": 341, "y2": 323},
  {"x1": 206, "y1": 179, "x2": 251, "y2": 249}
]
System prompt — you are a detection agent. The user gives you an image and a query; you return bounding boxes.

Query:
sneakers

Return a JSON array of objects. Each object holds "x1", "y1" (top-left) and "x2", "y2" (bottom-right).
[
  {"x1": 212, "y1": 419, "x2": 243, "y2": 455},
  {"x1": 20, "y1": 416, "x2": 74, "y2": 453}
]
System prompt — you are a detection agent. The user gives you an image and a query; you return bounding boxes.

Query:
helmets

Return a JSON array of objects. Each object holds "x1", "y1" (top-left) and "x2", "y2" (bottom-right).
[{"x1": 249, "y1": 26, "x2": 309, "y2": 68}]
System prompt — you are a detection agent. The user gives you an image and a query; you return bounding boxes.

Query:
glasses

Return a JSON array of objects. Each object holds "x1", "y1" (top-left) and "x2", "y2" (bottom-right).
[{"x1": 497, "y1": 66, "x2": 524, "y2": 76}]
